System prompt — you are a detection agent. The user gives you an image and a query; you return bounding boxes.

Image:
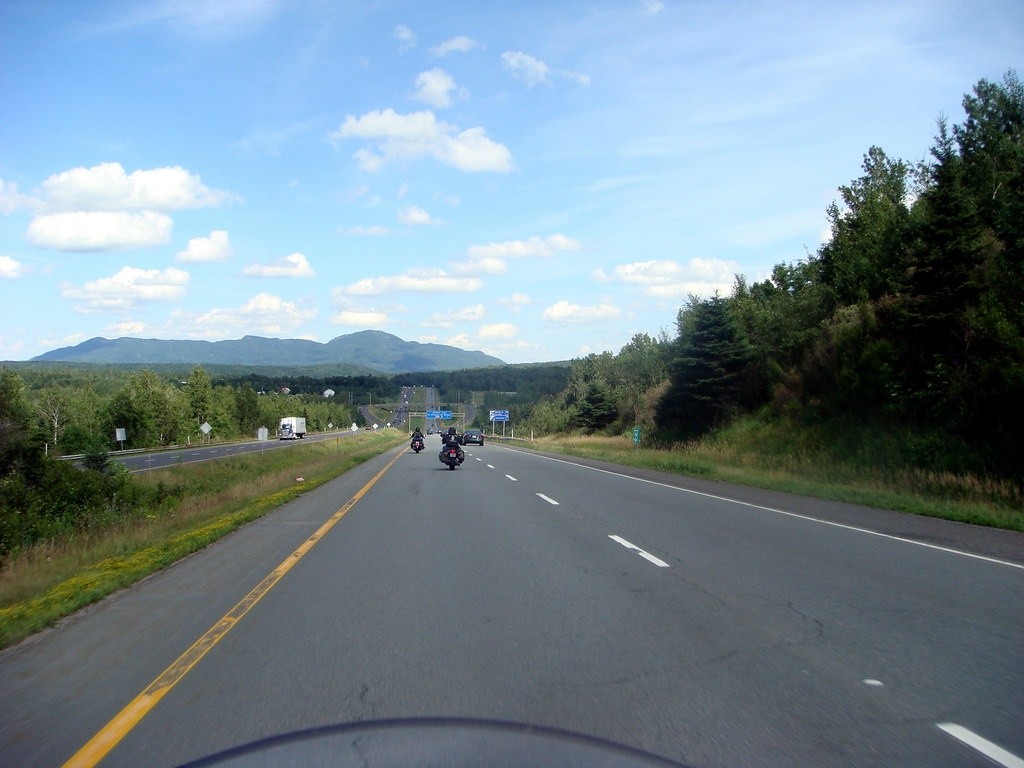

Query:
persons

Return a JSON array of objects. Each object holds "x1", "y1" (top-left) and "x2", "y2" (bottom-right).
[
  {"x1": 410, "y1": 427, "x2": 426, "y2": 449},
  {"x1": 439, "y1": 427, "x2": 465, "y2": 466}
]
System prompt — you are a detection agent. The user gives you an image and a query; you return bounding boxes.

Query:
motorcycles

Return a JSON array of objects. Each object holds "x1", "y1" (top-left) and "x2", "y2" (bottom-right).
[
  {"x1": 409, "y1": 434, "x2": 427, "y2": 454},
  {"x1": 440, "y1": 435, "x2": 465, "y2": 470}
]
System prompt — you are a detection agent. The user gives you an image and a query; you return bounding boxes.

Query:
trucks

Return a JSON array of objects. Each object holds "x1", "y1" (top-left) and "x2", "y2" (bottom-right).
[{"x1": 276, "y1": 417, "x2": 307, "y2": 440}]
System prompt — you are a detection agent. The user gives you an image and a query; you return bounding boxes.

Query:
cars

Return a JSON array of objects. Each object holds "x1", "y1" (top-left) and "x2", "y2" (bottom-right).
[
  {"x1": 405, "y1": 401, "x2": 408, "y2": 404},
  {"x1": 427, "y1": 429, "x2": 433, "y2": 434},
  {"x1": 461, "y1": 429, "x2": 485, "y2": 445},
  {"x1": 396, "y1": 417, "x2": 398, "y2": 420},
  {"x1": 435, "y1": 429, "x2": 448, "y2": 436},
  {"x1": 404, "y1": 394, "x2": 406, "y2": 398}
]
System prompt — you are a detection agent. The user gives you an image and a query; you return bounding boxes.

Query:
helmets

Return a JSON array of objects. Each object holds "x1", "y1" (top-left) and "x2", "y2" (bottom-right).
[
  {"x1": 449, "y1": 428, "x2": 455, "y2": 435},
  {"x1": 416, "y1": 427, "x2": 420, "y2": 432}
]
